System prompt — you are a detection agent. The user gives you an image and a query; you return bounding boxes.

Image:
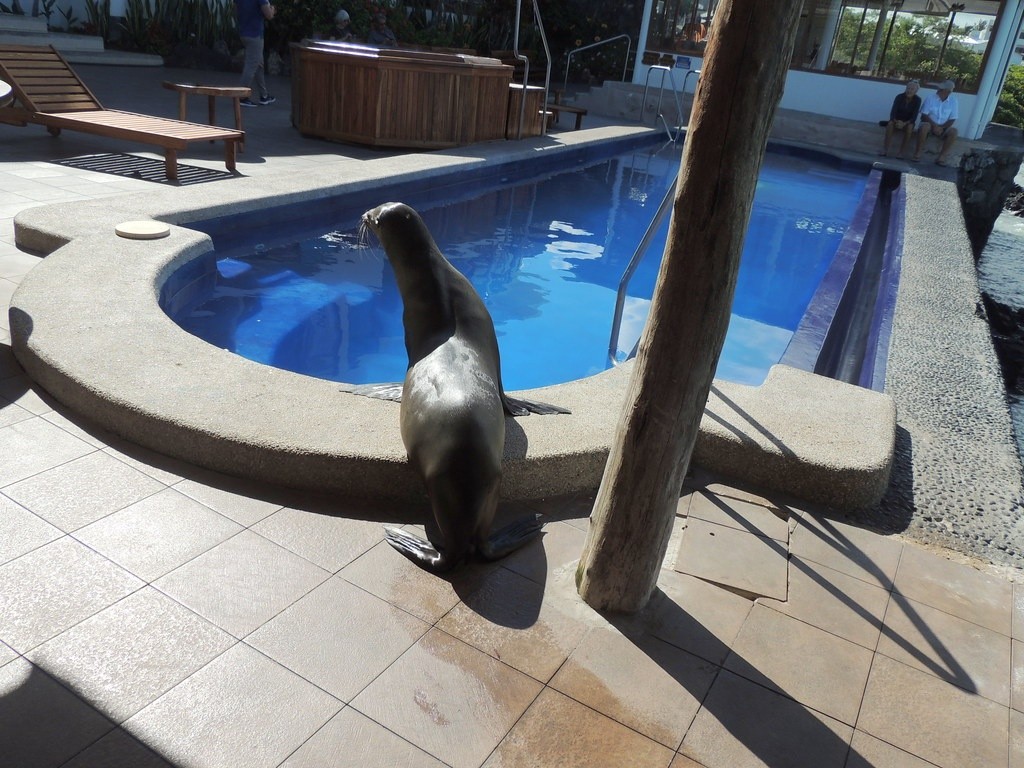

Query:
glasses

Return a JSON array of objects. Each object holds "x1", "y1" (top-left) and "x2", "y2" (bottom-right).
[
  {"x1": 344, "y1": 20, "x2": 350, "y2": 23},
  {"x1": 909, "y1": 79, "x2": 918, "y2": 85}
]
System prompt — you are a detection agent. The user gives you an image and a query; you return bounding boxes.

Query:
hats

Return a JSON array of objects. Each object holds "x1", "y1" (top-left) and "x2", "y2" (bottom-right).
[
  {"x1": 938, "y1": 79, "x2": 955, "y2": 90},
  {"x1": 336, "y1": 10, "x2": 349, "y2": 19}
]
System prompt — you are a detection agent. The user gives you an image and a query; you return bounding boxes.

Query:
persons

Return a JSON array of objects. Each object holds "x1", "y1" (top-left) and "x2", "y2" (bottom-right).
[
  {"x1": 809, "y1": 37, "x2": 820, "y2": 64},
  {"x1": 368, "y1": 13, "x2": 398, "y2": 48},
  {"x1": 879, "y1": 81, "x2": 921, "y2": 158},
  {"x1": 329, "y1": 10, "x2": 353, "y2": 42},
  {"x1": 911, "y1": 81, "x2": 958, "y2": 167},
  {"x1": 235, "y1": 0, "x2": 276, "y2": 107}
]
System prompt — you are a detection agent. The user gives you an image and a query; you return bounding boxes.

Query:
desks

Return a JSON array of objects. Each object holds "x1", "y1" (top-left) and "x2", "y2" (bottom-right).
[{"x1": 162, "y1": 78, "x2": 252, "y2": 153}]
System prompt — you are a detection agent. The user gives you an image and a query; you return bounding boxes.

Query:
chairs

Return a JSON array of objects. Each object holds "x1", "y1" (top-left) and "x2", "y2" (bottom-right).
[{"x1": 0, "y1": 44, "x2": 247, "y2": 183}]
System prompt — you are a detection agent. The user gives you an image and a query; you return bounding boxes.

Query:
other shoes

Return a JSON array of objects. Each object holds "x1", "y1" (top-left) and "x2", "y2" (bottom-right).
[
  {"x1": 240, "y1": 98, "x2": 258, "y2": 107},
  {"x1": 911, "y1": 153, "x2": 920, "y2": 162},
  {"x1": 880, "y1": 152, "x2": 888, "y2": 156},
  {"x1": 896, "y1": 153, "x2": 904, "y2": 159},
  {"x1": 936, "y1": 161, "x2": 946, "y2": 166},
  {"x1": 259, "y1": 93, "x2": 275, "y2": 105}
]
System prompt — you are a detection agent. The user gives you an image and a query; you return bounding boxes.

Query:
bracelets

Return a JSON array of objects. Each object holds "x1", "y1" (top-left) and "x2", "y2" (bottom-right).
[
  {"x1": 906, "y1": 121, "x2": 908, "y2": 126},
  {"x1": 944, "y1": 128, "x2": 946, "y2": 131}
]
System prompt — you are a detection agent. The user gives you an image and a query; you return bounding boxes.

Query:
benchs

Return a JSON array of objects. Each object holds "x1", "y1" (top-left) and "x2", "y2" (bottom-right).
[
  {"x1": 310, "y1": 33, "x2": 552, "y2": 83},
  {"x1": 541, "y1": 87, "x2": 587, "y2": 129}
]
